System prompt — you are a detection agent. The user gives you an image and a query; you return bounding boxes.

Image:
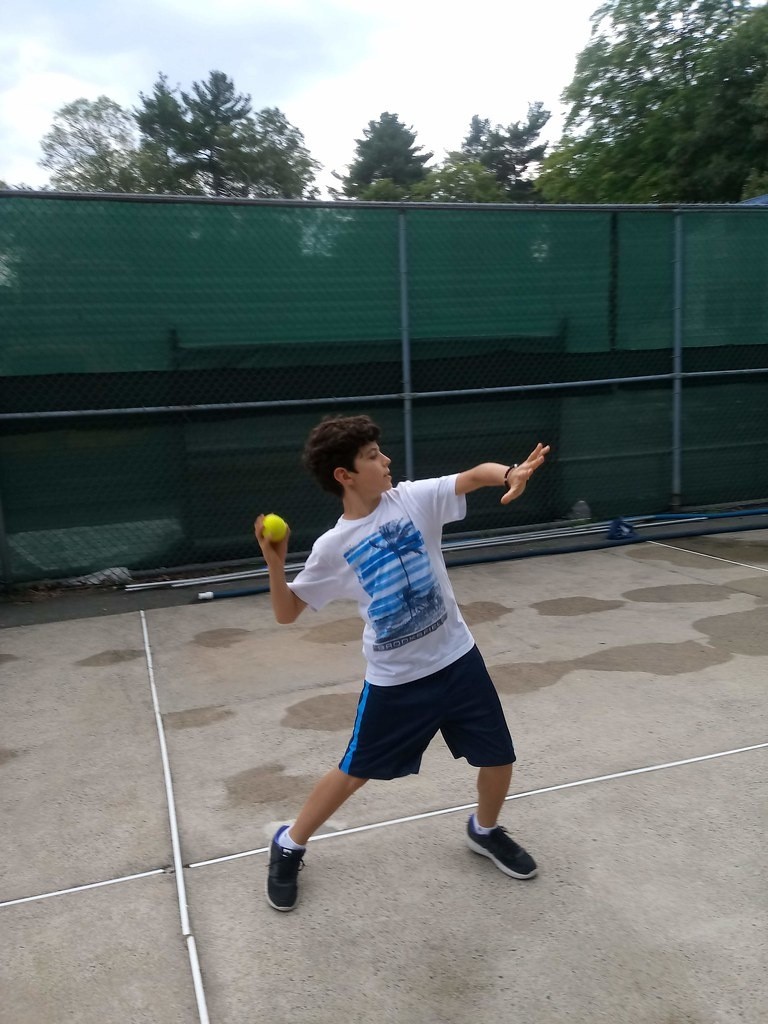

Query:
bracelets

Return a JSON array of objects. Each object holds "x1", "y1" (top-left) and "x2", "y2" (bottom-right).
[{"x1": 504, "y1": 464, "x2": 518, "y2": 488}]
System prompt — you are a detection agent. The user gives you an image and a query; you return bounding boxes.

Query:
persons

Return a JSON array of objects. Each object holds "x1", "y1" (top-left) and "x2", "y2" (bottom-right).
[{"x1": 253, "y1": 417, "x2": 551, "y2": 912}]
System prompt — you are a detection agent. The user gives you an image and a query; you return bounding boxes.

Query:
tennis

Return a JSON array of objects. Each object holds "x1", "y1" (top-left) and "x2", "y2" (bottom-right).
[{"x1": 260, "y1": 514, "x2": 287, "y2": 542}]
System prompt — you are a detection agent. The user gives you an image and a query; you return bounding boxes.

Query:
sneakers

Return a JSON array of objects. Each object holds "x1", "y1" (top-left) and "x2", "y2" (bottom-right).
[
  {"x1": 266, "y1": 824, "x2": 307, "y2": 911},
  {"x1": 466, "y1": 812, "x2": 538, "y2": 880}
]
